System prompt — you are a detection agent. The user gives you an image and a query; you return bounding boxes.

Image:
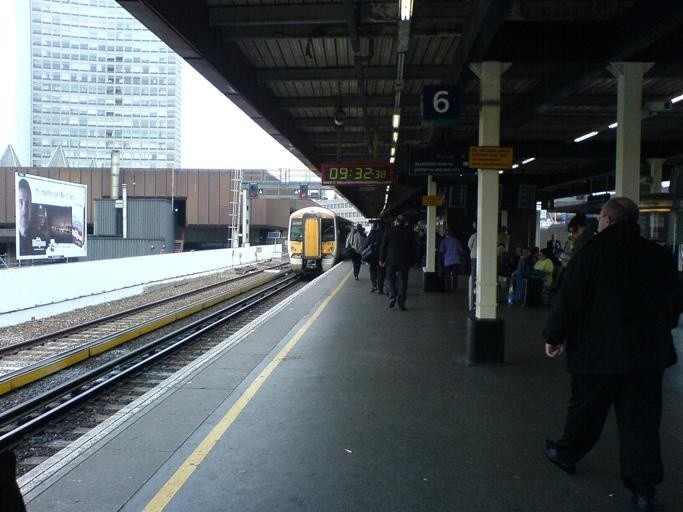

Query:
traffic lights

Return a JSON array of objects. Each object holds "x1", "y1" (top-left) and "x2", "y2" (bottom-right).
[
  {"x1": 249, "y1": 182, "x2": 259, "y2": 199},
  {"x1": 299, "y1": 184, "x2": 308, "y2": 199}
]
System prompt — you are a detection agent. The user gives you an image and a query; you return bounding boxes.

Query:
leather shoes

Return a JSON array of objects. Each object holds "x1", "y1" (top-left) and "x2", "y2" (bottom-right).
[
  {"x1": 543, "y1": 437, "x2": 576, "y2": 473},
  {"x1": 632, "y1": 496, "x2": 657, "y2": 512}
]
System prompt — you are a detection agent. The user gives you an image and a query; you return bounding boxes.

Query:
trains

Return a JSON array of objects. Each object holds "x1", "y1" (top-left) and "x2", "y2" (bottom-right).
[{"x1": 288, "y1": 206, "x2": 356, "y2": 273}]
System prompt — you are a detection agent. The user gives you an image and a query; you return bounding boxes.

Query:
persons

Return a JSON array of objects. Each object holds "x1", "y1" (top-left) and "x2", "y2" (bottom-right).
[
  {"x1": 539, "y1": 198, "x2": 682, "y2": 512},
  {"x1": 344, "y1": 212, "x2": 597, "y2": 312},
  {"x1": 19, "y1": 179, "x2": 34, "y2": 256}
]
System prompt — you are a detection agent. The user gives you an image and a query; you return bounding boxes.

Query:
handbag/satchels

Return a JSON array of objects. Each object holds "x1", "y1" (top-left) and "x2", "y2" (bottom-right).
[
  {"x1": 361, "y1": 244, "x2": 379, "y2": 263},
  {"x1": 340, "y1": 248, "x2": 355, "y2": 260}
]
